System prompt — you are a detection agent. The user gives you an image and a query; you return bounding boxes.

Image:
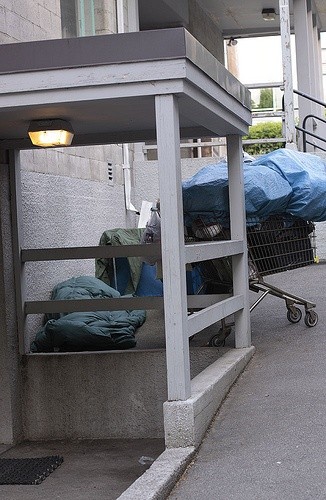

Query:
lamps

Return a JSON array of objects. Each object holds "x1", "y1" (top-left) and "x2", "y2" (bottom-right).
[
  {"x1": 261, "y1": 7, "x2": 276, "y2": 21},
  {"x1": 227, "y1": 37, "x2": 238, "y2": 47},
  {"x1": 27, "y1": 118, "x2": 75, "y2": 149}
]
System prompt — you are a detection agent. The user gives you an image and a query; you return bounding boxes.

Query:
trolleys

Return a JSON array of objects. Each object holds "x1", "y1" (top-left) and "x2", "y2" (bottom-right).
[{"x1": 150, "y1": 200, "x2": 321, "y2": 347}]
